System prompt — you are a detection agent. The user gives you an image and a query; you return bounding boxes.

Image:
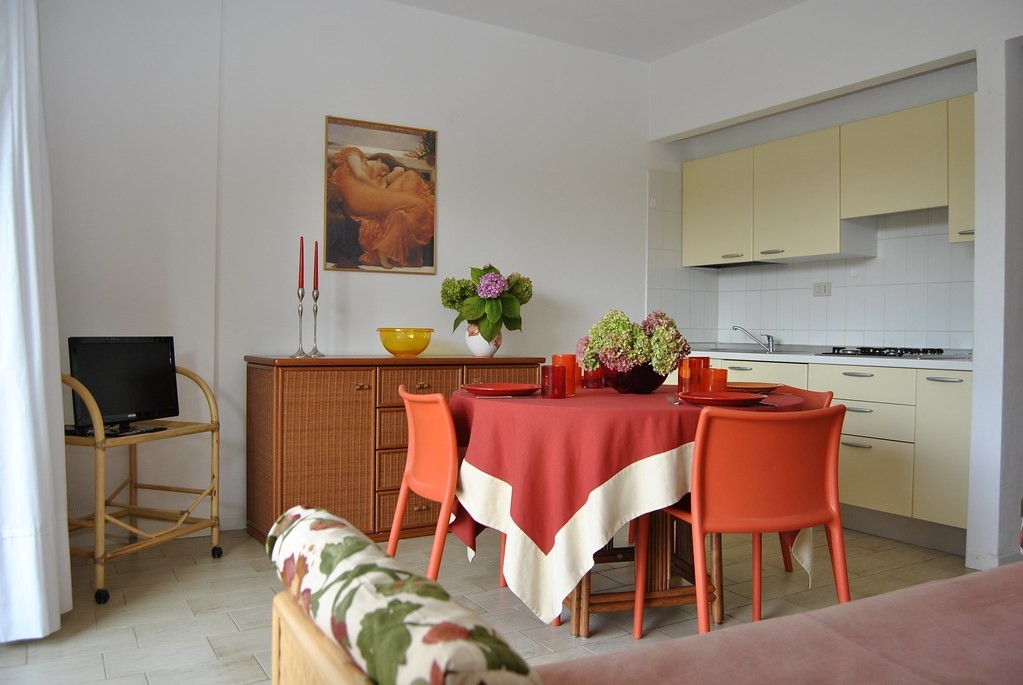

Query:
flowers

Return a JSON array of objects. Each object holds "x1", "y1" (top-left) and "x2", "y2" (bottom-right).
[
  {"x1": 440, "y1": 264, "x2": 533, "y2": 343},
  {"x1": 574, "y1": 310, "x2": 690, "y2": 375}
]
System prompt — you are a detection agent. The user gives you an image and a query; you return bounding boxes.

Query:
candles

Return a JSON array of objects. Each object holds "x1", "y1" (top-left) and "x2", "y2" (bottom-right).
[
  {"x1": 298, "y1": 236, "x2": 304, "y2": 288},
  {"x1": 313, "y1": 242, "x2": 318, "y2": 290}
]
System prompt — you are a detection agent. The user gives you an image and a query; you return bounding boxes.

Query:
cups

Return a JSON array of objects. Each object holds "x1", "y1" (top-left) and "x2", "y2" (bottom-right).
[
  {"x1": 539, "y1": 365, "x2": 566, "y2": 399},
  {"x1": 676, "y1": 357, "x2": 728, "y2": 395},
  {"x1": 550, "y1": 354, "x2": 582, "y2": 398}
]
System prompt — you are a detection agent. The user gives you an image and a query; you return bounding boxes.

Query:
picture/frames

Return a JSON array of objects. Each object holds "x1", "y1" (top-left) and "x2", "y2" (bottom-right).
[{"x1": 323, "y1": 115, "x2": 438, "y2": 275}]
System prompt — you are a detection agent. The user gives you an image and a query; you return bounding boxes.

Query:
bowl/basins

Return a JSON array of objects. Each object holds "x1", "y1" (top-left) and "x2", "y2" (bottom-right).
[{"x1": 377, "y1": 327, "x2": 435, "y2": 357}]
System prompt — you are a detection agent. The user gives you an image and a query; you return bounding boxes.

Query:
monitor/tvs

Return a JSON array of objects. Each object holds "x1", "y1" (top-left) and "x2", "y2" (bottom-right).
[{"x1": 68, "y1": 336, "x2": 180, "y2": 438}]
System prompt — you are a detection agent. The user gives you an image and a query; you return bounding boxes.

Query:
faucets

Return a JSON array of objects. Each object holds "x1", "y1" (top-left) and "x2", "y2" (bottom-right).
[{"x1": 732, "y1": 325, "x2": 775, "y2": 352}]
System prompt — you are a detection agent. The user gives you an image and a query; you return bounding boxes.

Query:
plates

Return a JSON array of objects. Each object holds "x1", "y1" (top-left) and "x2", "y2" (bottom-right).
[
  {"x1": 725, "y1": 382, "x2": 780, "y2": 393},
  {"x1": 461, "y1": 382, "x2": 543, "y2": 396},
  {"x1": 680, "y1": 393, "x2": 765, "y2": 406}
]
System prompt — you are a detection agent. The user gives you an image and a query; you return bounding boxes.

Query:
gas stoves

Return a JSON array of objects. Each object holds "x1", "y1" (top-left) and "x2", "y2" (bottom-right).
[{"x1": 814, "y1": 345, "x2": 949, "y2": 359}]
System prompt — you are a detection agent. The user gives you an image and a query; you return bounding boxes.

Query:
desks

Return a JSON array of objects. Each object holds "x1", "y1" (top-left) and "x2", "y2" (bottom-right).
[{"x1": 443, "y1": 385, "x2": 816, "y2": 637}]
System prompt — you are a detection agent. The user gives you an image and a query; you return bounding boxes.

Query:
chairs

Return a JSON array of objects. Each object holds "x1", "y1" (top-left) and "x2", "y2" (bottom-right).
[{"x1": 389, "y1": 381, "x2": 852, "y2": 637}]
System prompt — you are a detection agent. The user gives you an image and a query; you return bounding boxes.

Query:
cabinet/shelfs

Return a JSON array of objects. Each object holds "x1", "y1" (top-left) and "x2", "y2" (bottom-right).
[
  {"x1": 242, "y1": 355, "x2": 546, "y2": 545},
  {"x1": 681, "y1": 93, "x2": 976, "y2": 269},
  {"x1": 59, "y1": 366, "x2": 223, "y2": 605},
  {"x1": 663, "y1": 359, "x2": 972, "y2": 558}
]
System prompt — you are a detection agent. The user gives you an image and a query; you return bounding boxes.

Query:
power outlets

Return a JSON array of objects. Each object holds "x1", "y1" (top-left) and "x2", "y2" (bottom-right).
[{"x1": 813, "y1": 282, "x2": 831, "y2": 296}]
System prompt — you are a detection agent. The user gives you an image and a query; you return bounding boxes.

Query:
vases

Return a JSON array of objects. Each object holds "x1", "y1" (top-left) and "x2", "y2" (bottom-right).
[
  {"x1": 599, "y1": 360, "x2": 669, "y2": 392},
  {"x1": 465, "y1": 323, "x2": 503, "y2": 357}
]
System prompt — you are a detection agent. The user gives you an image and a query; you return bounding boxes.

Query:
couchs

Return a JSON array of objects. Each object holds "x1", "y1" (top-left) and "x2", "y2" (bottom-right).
[{"x1": 265, "y1": 503, "x2": 1023, "y2": 685}]
